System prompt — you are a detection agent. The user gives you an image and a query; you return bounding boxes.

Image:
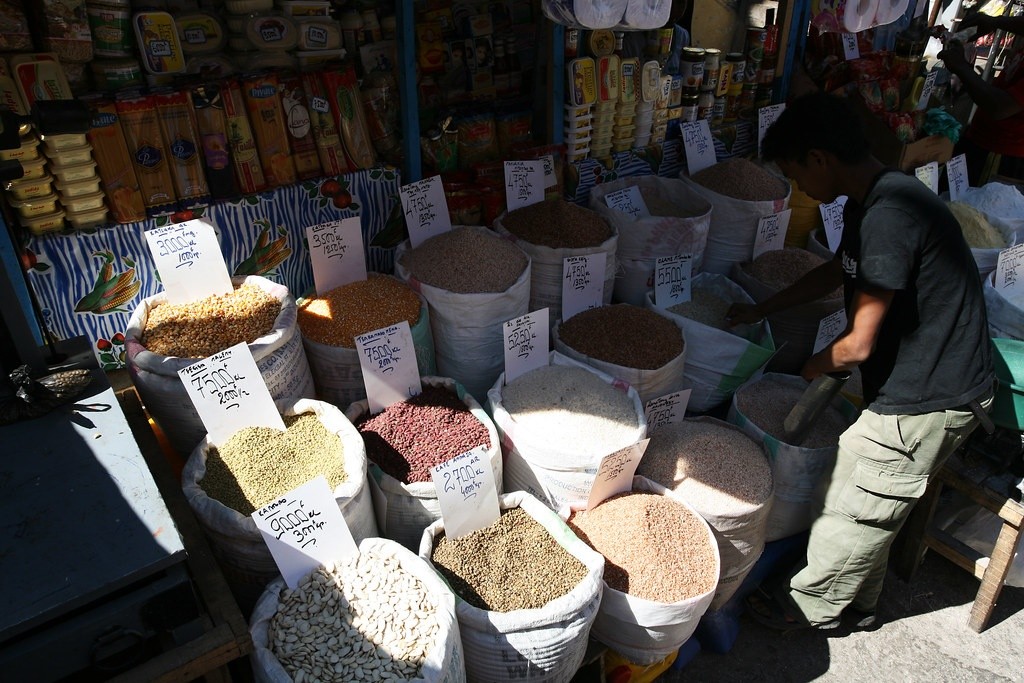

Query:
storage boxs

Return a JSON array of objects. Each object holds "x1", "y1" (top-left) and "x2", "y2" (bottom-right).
[
  {"x1": 632, "y1": 61, "x2": 682, "y2": 148},
  {"x1": 591, "y1": 111, "x2": 616, "y2": 123},
  {"x1": 612, "y1": 137, "x2": 635, "y2": 152},
  {"x1": 563, "y1": 102, "x2": 595, "y2": 119},
  {"x1": 589, "y1": 131, "x2": 613, "y2": 147},
  {"x1": 590, "y1": 121, "x2": 615, "y2": 136},
  {"x1": 563, "y1": 125, "x2": 592, "y2": 140},
  {"x1": 0, "y1": 0, "x2": 346, "y2": 236},
  {"x1": 619, "y1": 59, "x2": 637, "y2": 106},
  {"x1": 590, "y1": 99, "x2": 618, "y2": 113},
  {"x1": 590, "y1": 142, "x2": 612, "y2": 158},
  {"x1": 563, "y1": 137, "x2": 591, "y2": 151},
  {"x1": 565, "y1": 57, "x2": 597, "y2": 106},
  {"x1": 784, "y1": 57, "x2": 954, "y2": 175},
  {"x1": 596, "y1": 54, "x2": 621, "y2": 102},
  {"x1": 613, "y1": 123, "x2": 636, "y2": 139},
  {"x1": 564, "y1": 148, "x2": 590, "y2": 163},
  {"x1": 614, "y1": 112, "x2": 636, "y2": 127},
  {"x1": 615, "y1": 102, "x2": 639, "y2": 115},
  {"x1": 563, "y1": 112, "x2": 592, "y2": 129}
]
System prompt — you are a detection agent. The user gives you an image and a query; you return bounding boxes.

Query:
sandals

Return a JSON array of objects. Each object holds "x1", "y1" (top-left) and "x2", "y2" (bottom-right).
[
  {"x1": 747, "y1": 593, "x2": 841, "y2": 630},
  {"x1": 844, "y1": 610, "x2": 876, "y2": 626}
]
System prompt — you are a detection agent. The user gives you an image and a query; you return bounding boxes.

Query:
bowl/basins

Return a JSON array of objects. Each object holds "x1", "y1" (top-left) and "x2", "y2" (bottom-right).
[{"x1": 984, "y1": 338, "x2": 1024, "y2": 430}]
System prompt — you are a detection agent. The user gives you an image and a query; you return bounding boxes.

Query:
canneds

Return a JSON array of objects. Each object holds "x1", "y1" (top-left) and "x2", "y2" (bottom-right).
[{"x1": 679, "y1": 25, "x2": 780, "y2": 126}]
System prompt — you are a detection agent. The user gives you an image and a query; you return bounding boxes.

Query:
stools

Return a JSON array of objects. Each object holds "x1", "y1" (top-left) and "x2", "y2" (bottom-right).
[{"x1": 897, "y1": 423, "x2": 1024, "y2": 633}]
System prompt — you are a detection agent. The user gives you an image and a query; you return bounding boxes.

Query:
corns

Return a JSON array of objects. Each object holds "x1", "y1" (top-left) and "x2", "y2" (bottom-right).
[
  {"x1": 75, "y1": 253, "x2": 141, "y2": 313},
  {"x1": 231, "y1": 218, "x2": 293, "y2": 277}
]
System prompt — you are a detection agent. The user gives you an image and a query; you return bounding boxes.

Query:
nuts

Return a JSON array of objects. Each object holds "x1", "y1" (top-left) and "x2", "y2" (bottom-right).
[{"x1": 266, "y1": 550, "x2": 437, "y2": 683}]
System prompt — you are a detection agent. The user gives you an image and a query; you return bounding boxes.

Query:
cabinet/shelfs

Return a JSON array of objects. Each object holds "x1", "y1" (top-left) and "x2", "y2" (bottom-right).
[{"x1": 0, "y1": 336, "x2": 214, "y2": 683}]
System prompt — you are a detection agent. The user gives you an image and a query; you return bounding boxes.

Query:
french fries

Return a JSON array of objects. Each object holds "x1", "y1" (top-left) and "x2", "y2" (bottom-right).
[{"x1": 416, "y1": 113, "x2": 531, "y2": 168}]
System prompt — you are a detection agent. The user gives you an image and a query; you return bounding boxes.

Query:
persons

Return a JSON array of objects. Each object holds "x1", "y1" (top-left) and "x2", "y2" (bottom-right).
[
  {"x1": 622, "y1": 1, "x2": 690, "y2": 76},
  {"x1": 756, "y1": 105, "x2": 1001, "y2": 631},
  {"x1": 938, "y1": 14, "x2": 1024, "y2": 187}
]
